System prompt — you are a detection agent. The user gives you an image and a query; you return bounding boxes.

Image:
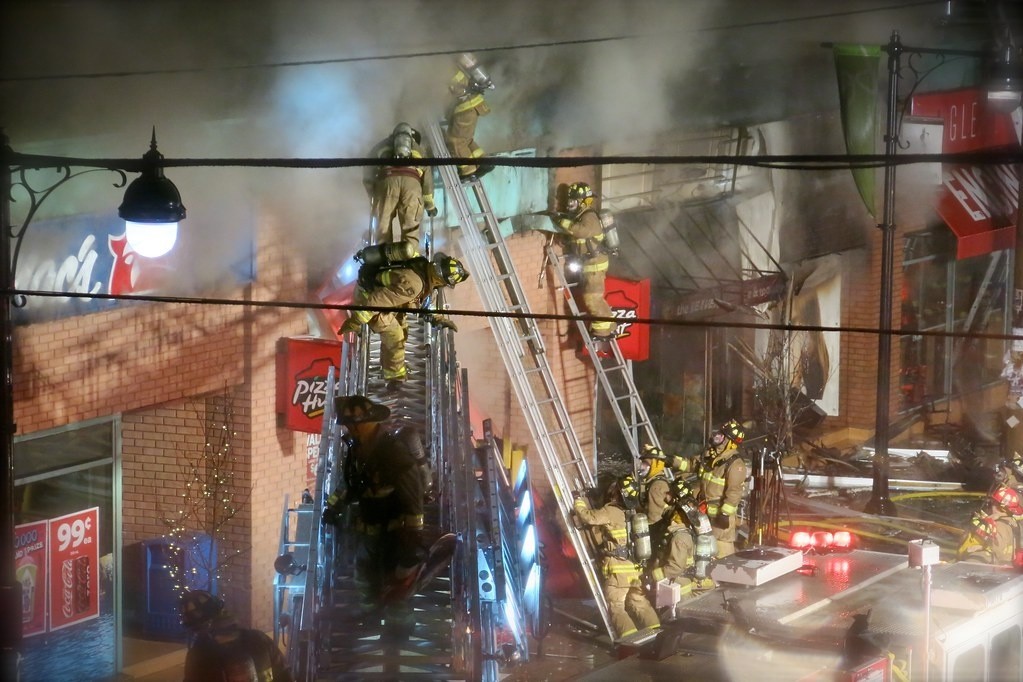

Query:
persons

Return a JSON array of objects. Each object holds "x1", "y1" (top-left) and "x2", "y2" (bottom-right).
[
  {"x1": 337, "y1": 241, "x2": 470, "y2": 393},
  {"x1": 557, "y1": 183, "x2": 618, "y2": 341},
  {"x1": 363, "y1": 122, "x2": 438, "y2": 248},
  {"x1": 179, "y1": 588, "x2": 287, "y2": 682},
  {"x1": 571, "y1": 443, "x2": 716, "y2": 637},
  {"x1": 672, "y1": 420, "x2": 747, "y2": 558},
  {"x1": 430, "y1": 51, "x2": 495, "y2": 181},
  {"x1": 955, "y1": 458, "x2": 1023, "y2": 566},
  {"x1": 323, "y1": 396, "x2": 428, "y2": 644}
]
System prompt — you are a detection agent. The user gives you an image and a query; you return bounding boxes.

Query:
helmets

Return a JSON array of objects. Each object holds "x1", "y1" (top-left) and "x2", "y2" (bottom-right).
[
  {"x1": 412, "y1": 128, "x2": 420, "y2": 144},
  {"x1": 720, "y1": 421, "x2": 745, "y2": 446},
  {"x1": 433, "y1": 252, "x2": 466, "y2": 289},
  {"x1": 180, "y1": 589, "x2": 223, "y2": 626},
  {"x1": 639, "y1": 447, "x2": 667, "y2": 459},
  {"x1": 678, "y1": 498, "x2": 701, "y2": 527},
  {"x1": 566, "y1": 182, "x2": 597, "y2": 199},
  {"x1": 336, "y1": 394, "x2": 390, "y2": 424},
  {"x1": 991, "y1": 487, "x2": 1023, "y2": 515},
  {"x1": 617, "y1": 475, "x2": 640, "y2": 509}
]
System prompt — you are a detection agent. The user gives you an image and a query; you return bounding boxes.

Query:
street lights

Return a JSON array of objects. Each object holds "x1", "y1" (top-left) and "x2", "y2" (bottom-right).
[
  {"x1": 0, "y1": 124, "x2": 186, "y2": 682},
  {"x1": 863, "y1": 29, "x2": 1022, "y2": 517}
]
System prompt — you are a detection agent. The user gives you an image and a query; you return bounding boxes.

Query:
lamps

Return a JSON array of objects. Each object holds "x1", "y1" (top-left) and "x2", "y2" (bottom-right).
[
  {"x1": 118, "y1": 126, "x2": 186, "y2": 257},
  {"x1": 788, "y1": 530, "x2": 854, "y2": 554}
]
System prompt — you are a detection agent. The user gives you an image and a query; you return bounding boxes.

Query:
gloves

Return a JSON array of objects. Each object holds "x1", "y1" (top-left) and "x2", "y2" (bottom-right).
[
  {"x1": 432, "y1": 314, "x2": 458, "y2": 332},
  {"x1": 337, "y1": 318, "x2": 362, "y2": 337},
  {"x1": 428, "y1": 207, "x2": 438, "y2": 217},
  {"x1": 717, "y1": 513, "x2": 729, "y2": 530},
  {"x1": 572, "y1": 490, "x2": 581, "y2": 498}
]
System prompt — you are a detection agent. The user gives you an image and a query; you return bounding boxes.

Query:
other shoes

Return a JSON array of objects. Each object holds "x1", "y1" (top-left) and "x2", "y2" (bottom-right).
[
  {"x1": 388, "y1": 381, "x2": 401, "y2": 391},
  {"x1": 593, "y1": 335, "x2": 615, "y2": 342},
  {"x1": 383, "y1": 623, "x2": 413, "y2": 642},
  {"x1": 475, "y1": 164, "x2": 494, "y2": 177},
  {"x1": 459, "y1": 174, "x2": 477, "y2": 182}
]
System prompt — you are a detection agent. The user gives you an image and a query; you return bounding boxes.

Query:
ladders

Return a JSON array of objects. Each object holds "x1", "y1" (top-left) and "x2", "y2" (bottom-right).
[
  {"x1": 426, "y1": 117, "x2": 665, "y2": 646},
  {"x1": 543, "y1": 245, "x2": 664, "y2": 466}
]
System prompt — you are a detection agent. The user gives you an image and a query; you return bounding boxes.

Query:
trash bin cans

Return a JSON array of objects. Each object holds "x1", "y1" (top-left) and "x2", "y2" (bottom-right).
[{"x1": 141, "y1": 532, "x2": 218, "y2": 641}]
